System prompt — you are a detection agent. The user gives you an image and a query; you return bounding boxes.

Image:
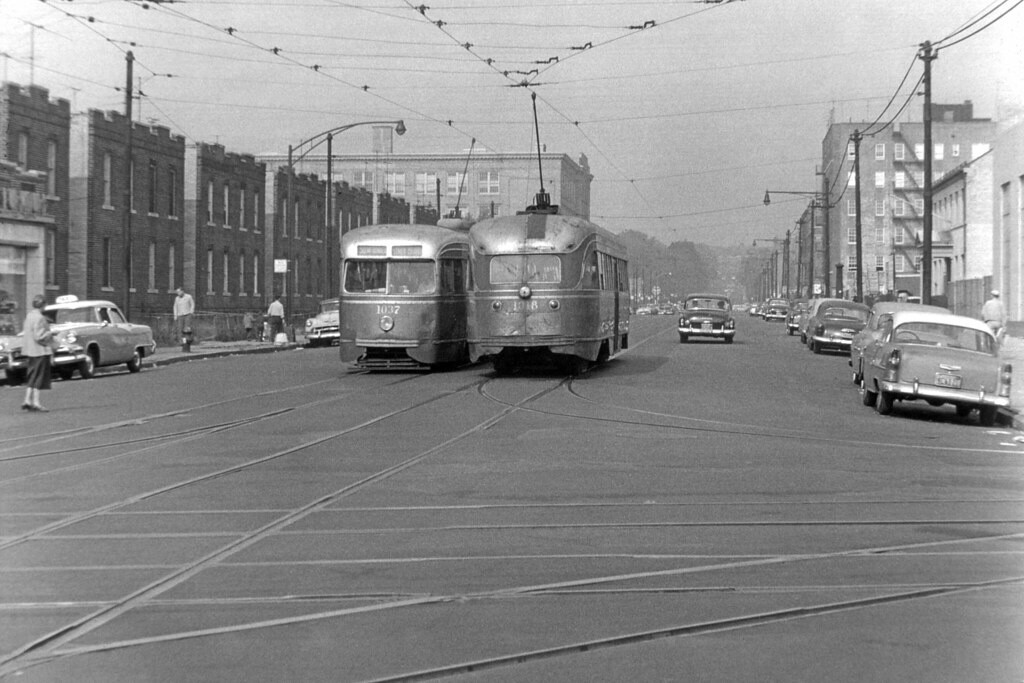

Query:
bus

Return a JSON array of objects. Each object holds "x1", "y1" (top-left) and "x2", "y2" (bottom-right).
[
  {"x1": 339, "y1": 219, "x2": 471, "y2": 371},
  {"x1": 465, "y1": 214, "x2": 630, "y2": 375}
]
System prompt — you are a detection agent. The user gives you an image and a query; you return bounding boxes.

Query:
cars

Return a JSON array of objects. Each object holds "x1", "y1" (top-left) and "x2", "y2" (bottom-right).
[
  {"x1": 763, "y1": 299, "x2": 791, "y2": 322},
  {"x1": 785, "y1": 298, "x2": 843, "y2": 344},
  {"x1": 806, "y1": 301, "x2": 870, "y2": 354},
  {"x1": 862, "y1": 312, "x2": 1013, "y2": 426},
  {"x1": 750, "y1": 303, "x2": 768, "y2": 320},
  {"x1": 1, "y1": 295, "x2": 157, "y2": 386},
  {"x1": 850, "y1": 302, "x2": 953, "y2": 384},
  {"x1": 678, "y1": 294, "x2": 736, "y2": 344},
  {"x1": 304, "y1": 297, "x2": 340, "y2": 347}
]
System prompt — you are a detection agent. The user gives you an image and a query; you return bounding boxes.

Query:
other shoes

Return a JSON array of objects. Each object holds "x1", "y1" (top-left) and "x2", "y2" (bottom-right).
[{"x1": 22, "y1": 403, "x2": 49, "y2": 412}]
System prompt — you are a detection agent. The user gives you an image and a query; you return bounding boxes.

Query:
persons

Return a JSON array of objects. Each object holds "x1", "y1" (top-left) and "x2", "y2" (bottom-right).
[
  {"x1": 981, "y1": 290, "x2": 1005, "y2": 331},
  {"x1": 267, "y1": 294, "x2": 284, "y2": 341},
  {"x1": 717, "y1": 301, "x2": 725, "y2": 310},
  {"x1": 20, "y1": 294, "x2": 59, "y2": 413},
  {"x1": 255, "y1": 312, "x2": 267, "y2": 342},
  {"x1": 243, "y1": 311, "x2": 255, "y2": 341},
  {"x1": 897, "y1": 293, "x2": 908, "y2": 303},
  {"x1": 173, "y1": 287, "x2": 195, "y2": 347}
]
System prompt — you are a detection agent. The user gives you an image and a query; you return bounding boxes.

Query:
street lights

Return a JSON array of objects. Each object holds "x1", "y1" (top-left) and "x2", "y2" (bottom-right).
[
  {"x1": 753, "y1": 230, "x2": 791, "y2": 300},
  {"x1": 764, "y1": 178, "x2": 830, "y2": 297},
  {"x1": 287, "y1": 120, "x2": 407, "y2": 342}
]
